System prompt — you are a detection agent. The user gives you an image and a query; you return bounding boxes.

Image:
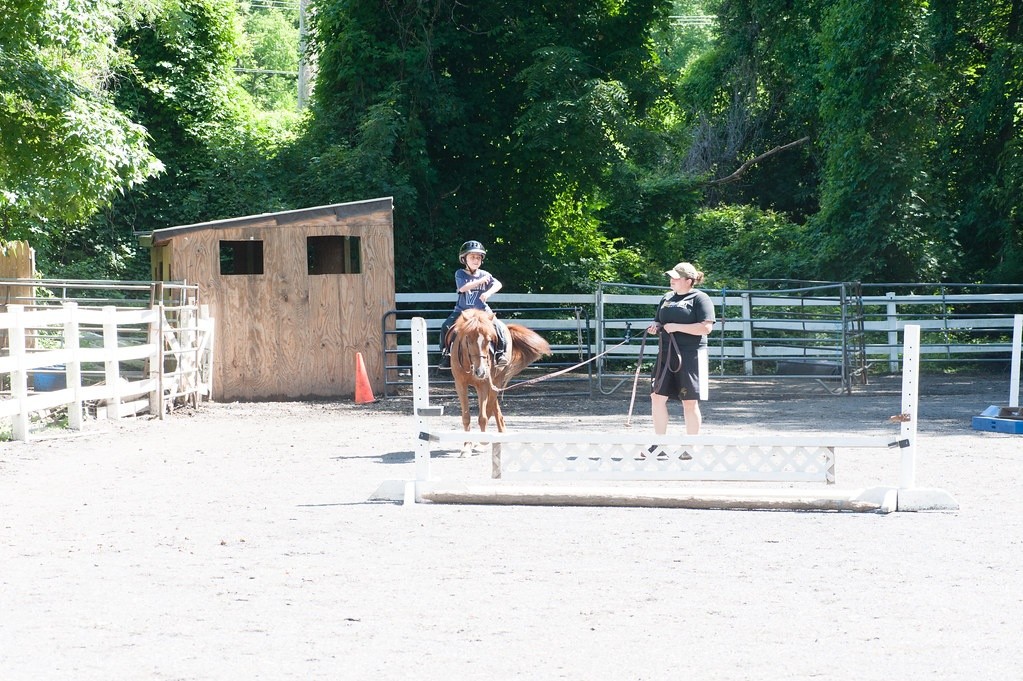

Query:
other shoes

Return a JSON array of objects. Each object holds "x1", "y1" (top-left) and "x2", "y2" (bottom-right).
[
  {"x1": 495, "y1": 353, "x2": 508, "y2": 365},
  {"x1": 680, "y1": 451, "x2": 692, "y2": 459},
  {"x1": 641, "y1": 445, "x2": 666, "y2": 456},
  {"x1": 440, "y1": 355, "x2": 451, "y2": 368}
]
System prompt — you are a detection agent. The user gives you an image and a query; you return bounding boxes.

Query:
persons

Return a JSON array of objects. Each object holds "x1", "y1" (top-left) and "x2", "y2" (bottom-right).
[
  {"x1": 438, "y1": 240, "x2": 506, "y2": 370},
  {"x1": 639, "y1": 263, "x2": 717, "y2": 460}
]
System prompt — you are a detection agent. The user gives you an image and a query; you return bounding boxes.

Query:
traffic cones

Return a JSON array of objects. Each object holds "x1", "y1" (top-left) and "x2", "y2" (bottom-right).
[{"x1": 353, "y1": 353, "x2": 379, "y2": 406}]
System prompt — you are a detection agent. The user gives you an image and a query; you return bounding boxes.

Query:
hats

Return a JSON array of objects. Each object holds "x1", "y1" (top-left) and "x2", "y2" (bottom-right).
[{"x1": 665, "y1": 262, "x2": 697, "y2": 280}]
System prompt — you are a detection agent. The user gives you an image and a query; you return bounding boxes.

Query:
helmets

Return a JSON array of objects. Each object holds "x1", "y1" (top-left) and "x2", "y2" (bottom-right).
[{"x1": 459, "y1": 240, "x2": 486, "y2": 264}]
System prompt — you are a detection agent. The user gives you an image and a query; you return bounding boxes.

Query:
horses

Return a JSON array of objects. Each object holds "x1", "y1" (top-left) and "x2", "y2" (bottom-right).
[{"x1": 450, "y1": 308, "x2": 551, "y2": 458}]
